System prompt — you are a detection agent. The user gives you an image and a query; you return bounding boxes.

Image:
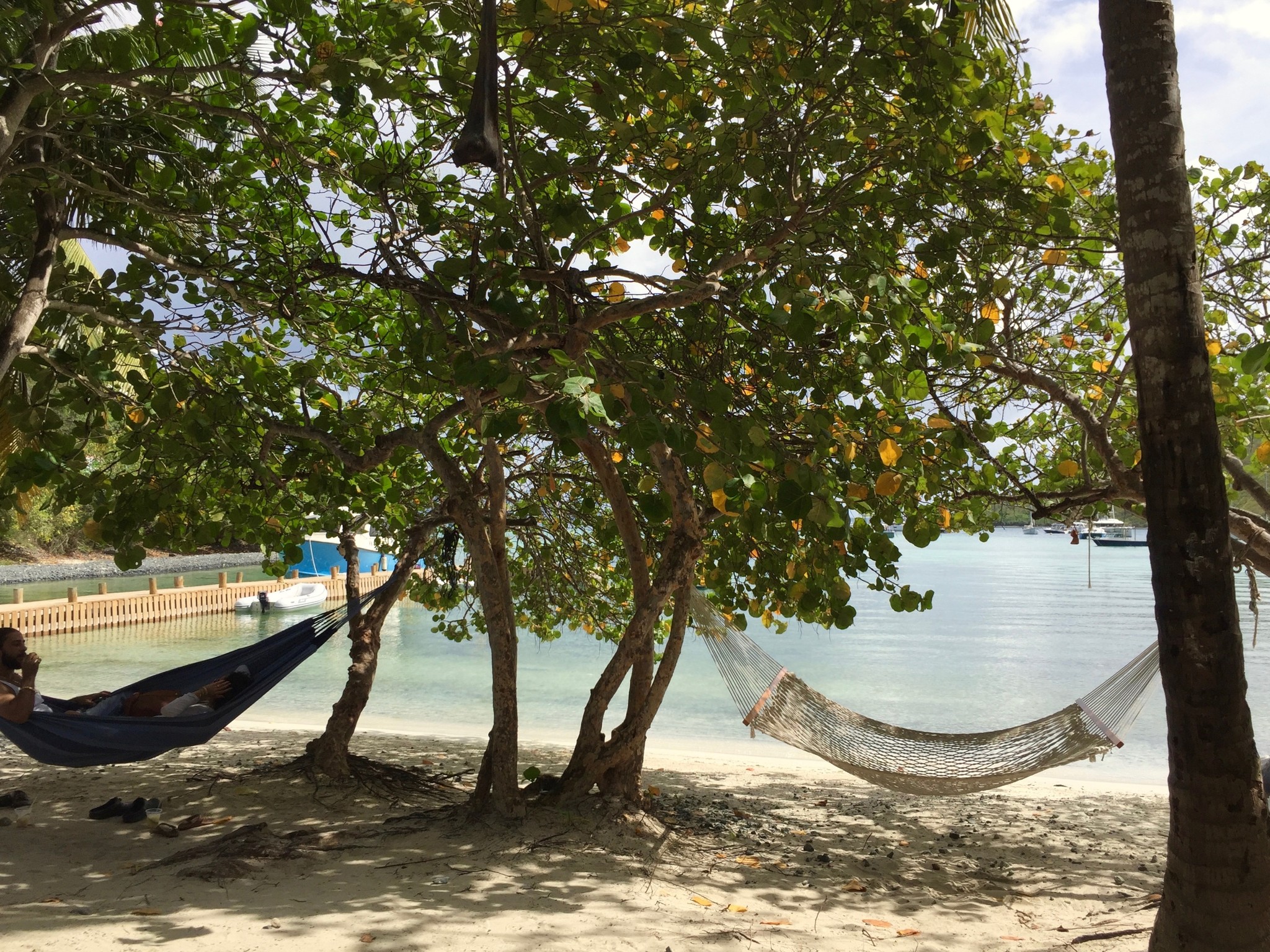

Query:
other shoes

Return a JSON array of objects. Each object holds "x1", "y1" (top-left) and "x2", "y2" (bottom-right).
[
  {"x1": 12, "y1": 790, "x2": 30, "y2": 808},
  {"x1": 0, "y1": 794, "x2": 13, "y2": 807}
]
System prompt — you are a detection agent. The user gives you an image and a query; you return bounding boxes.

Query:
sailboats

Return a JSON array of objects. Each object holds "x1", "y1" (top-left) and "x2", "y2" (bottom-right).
[{"x1": 1021, "y1": 505, "x2": 1040, "y2": 535}]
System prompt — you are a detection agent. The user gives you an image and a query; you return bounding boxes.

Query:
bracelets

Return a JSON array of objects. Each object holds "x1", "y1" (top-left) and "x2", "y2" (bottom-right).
[
  {"x1": 200, "y1": 686, "x2": 208, "y2": 699},
  {"x1": 19, "y1": 686, "x2": 37, "y2": 693}
]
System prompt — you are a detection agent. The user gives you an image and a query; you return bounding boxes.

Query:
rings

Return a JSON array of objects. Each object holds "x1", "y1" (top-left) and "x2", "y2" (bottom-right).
[
  {"x1": 217, "y1": 685, "x2": 220, "y2": 687},
  {"x1": 37, "y1": 660, "x2": 40, "y2": 663}
]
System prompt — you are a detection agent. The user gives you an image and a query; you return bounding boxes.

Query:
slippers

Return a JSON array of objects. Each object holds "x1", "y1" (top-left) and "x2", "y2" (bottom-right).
[
  {"x1": 88, "y1": 797, "x2": 132, "y2": 819},
  {"x1": 122, "y1": 797, "x2": 162, "y2": 823},
  {"x1": 178, "y1": 814, "x2": 215, "y2": 830},
  {"x1": 153, "y1": 822, "x2": 180, "y2": 838}
]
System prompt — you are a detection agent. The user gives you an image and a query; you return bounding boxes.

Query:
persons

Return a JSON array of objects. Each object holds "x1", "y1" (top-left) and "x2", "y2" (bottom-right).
[
  {"x1": 118, "y1": 663, "x2": 253, "y2": 762},
  {"x1": 0, "y1": 626, "x2": 134, "y2": 768}
]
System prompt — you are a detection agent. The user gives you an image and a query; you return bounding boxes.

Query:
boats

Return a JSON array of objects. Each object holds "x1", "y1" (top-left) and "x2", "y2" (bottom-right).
[
  {"x1": 884, "y1": 531, "x2": 897, "y2": 539},
  {"x1": 1091, "y1": 527, "x2": 1149, "y2": 547},
  {"x1": 1079, "y1": 527, "x2": 1106, "y2": 539},
  {"x1": 276, "y1": 531, "x2": 429, "y2": 580},
  {"x1": 234, "y1": 582, "x2": 328, "y2": 612},
  {"x1": 1043, "y1": 523, "x2": 1069, "y2": 534}
]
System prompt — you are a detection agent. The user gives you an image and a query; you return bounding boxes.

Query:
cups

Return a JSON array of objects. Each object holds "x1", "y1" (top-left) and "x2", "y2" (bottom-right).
[
  {"x1": 146, "y1": 808, "x2": 162, "y2": 828},
  {"x1": 14, "y1": 808, "x2": 32, "y2": 826}
]
